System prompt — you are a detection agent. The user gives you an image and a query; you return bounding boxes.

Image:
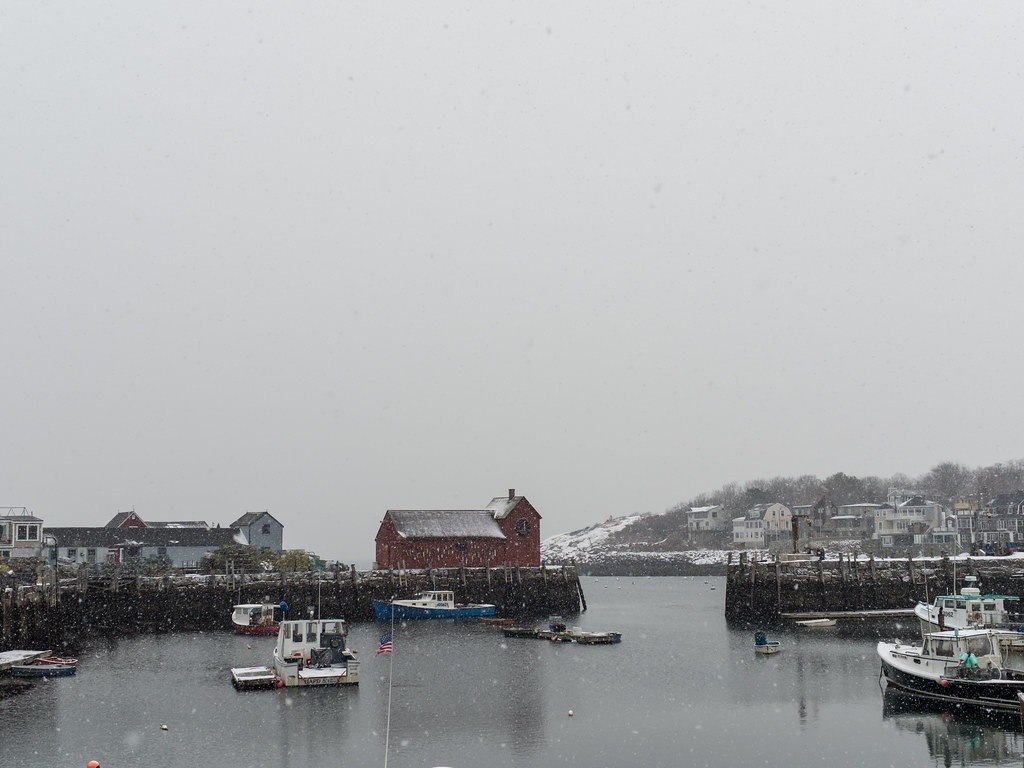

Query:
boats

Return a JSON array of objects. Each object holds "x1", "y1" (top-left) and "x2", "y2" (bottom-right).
[
  {"x1": 876, "y1": 559, "x2": 1024, "y2": 713},
  {"x1": 753, "y1": 631, "x2": 781, "y2": 656},
  {"x1": 231, "y1": 603, "x2": 292, "y2": 636},
  {"x1": 501, "y1": 614, "x2": 622, "y2": 644},
  {"x1": 273, "y1": 570, "x2": 362, "y2": 690},
  {"x1": 33, "y1": 657, "x2": 79, "y2": 666},
  {"x1": 372, "y1": 590, "x2": 497, "y2": 620},
  {"x1": 11, "y1": 664, "x2": 76, "y2": 677},
  {"x1": 913, "y1": 538, "x2": 1024, "y2": 640},
  {"x1": 795, "y1": 618, "x2": 838, "y2": 628}
]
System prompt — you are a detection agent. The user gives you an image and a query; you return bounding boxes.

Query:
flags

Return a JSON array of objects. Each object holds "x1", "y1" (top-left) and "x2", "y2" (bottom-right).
[{"x1": 375, "y1": 631, "x2": 393, "y2": 657}]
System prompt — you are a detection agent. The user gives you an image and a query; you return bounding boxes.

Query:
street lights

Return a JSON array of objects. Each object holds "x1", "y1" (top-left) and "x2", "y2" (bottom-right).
[{"x1": 39, "y1": 536, "x2": 61, "y2": 612}]
